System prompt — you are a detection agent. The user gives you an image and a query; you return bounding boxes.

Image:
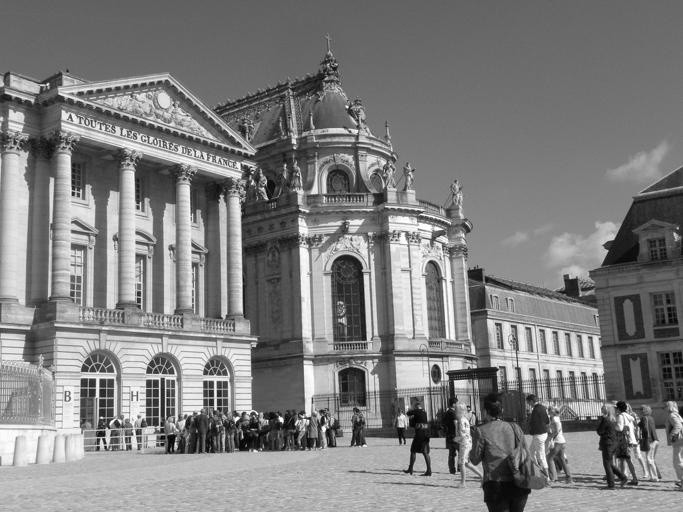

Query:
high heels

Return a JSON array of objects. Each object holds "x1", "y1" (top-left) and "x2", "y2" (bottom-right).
[{"x1": 403, "y1": 469, "x2": 413, "y2": 475}]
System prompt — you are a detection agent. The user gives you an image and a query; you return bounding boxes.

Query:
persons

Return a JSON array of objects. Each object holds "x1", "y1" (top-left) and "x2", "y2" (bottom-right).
[
  {"x1": 468, "y1": 395, "x2": 531, "y2": 512},
  {"x1": 403, "y1": 403, "x2": 432, "y2": 477},
  {"x1": 443, "y1": 393, "x2": 682, "y2": 489},
  {"x1": 83, "y1": 407, "x2": 366, "y2": 454},
  {"x1": 394, "y1": 409, "x2": 408, "y2": 445}
]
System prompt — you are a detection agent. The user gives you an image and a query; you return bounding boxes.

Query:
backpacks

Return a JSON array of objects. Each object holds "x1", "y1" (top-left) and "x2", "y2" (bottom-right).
[
  {"x1": 334, "y1": 418, "x2": 341, "y2": 429},
  {"x1": 359, "y1": 414, "x2": 365, "y2": 426}
]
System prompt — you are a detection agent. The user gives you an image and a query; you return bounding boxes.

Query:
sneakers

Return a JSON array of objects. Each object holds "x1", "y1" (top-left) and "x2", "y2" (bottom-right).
[
  {"x1": 399, "y1": 440, "x2": 406, "y2": 445},
  {"x1": 602, "y1": 471, "x2": 683, "y2": 493},
  {"x1": 165, "y1": 443, "x2": 368, "y2": 455},
  {"x1": 424, "y1": 472, "x2": 432, "y2": 476},
  {"x1": 449, "y1": 467, "x2": 484, "y2": 488}
]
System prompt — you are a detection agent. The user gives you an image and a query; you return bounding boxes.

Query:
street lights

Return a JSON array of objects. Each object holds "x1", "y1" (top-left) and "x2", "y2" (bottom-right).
[
  {"x1": 506, "y1": 333, "x2": 524, "y2": 423},
  {"x1": 419, "y1": 343, "x2": 433, "y2": 424}
]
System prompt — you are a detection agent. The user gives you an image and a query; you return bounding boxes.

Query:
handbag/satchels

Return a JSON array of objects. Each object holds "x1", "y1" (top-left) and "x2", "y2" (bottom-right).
[
  {"x1": 505, "y1": 422, "x2": 549, "y2": 490},
  {"x1": 666, "y1": 422, "x2": 683, "y2": 446},
  {"x1": 616, "y1": 430, "x2": 630, "y2": 459},
  {"x1": 640, "y1": 438, "x2": 651, "y2": 451}
]
